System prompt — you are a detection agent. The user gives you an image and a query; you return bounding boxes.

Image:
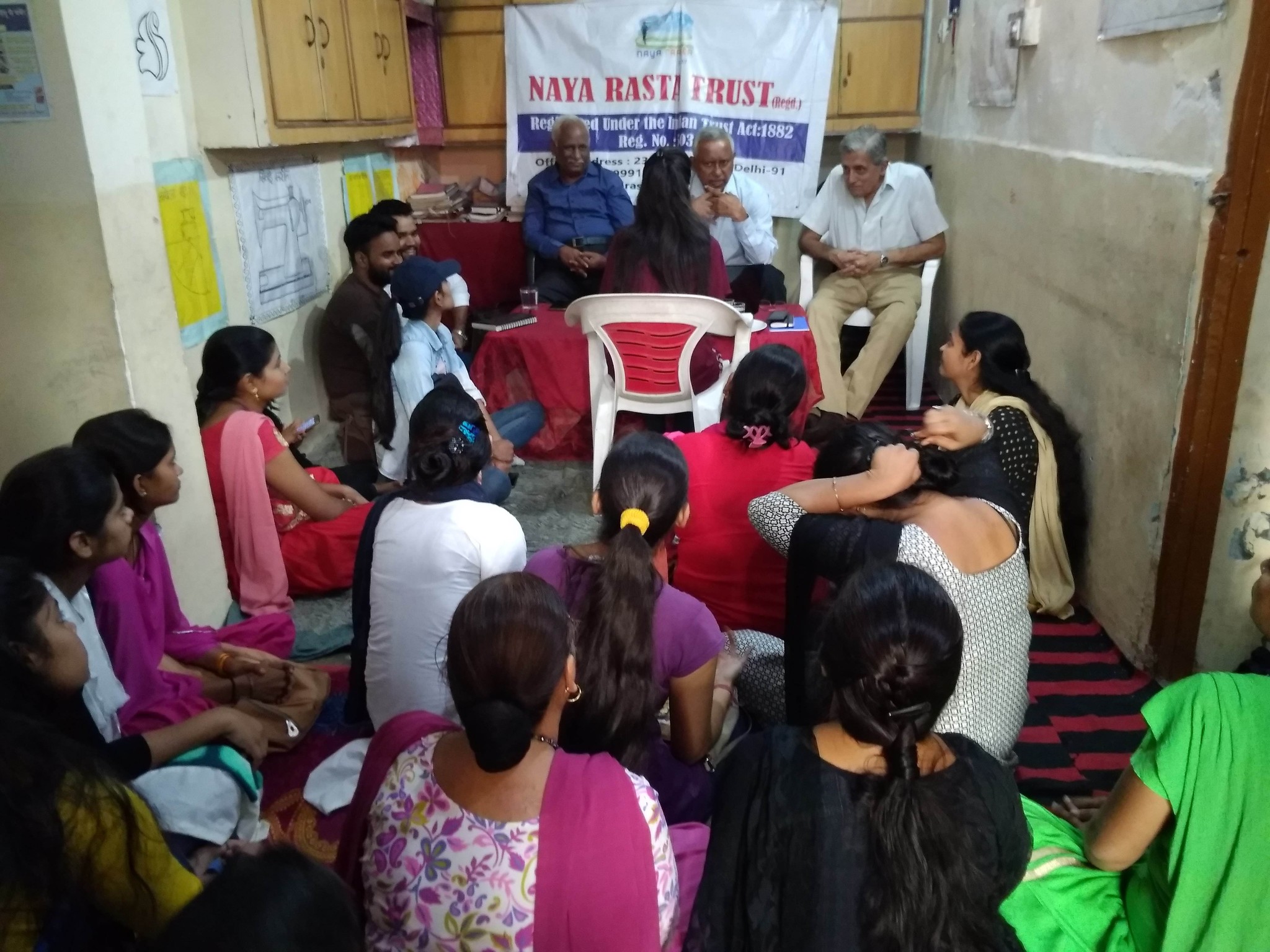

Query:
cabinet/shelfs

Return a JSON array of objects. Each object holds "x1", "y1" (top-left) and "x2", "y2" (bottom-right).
[
  {"x1": 179, "y1": 0, "x2": 417, "y2": 152},
  {"x1": 432, "y1": 0, "x2": 927, "y2": 151}
]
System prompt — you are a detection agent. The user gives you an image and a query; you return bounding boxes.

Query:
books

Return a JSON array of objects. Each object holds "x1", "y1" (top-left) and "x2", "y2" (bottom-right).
[{"x1": 407, "y1": 180, "x2": 507, "y2": 224}]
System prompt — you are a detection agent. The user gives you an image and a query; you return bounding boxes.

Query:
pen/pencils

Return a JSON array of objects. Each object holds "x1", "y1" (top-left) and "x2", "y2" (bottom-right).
[
  {"x1": 880, "y1": 216, "x2": 883, "y2": 229},
  {"x1": 787, "y1": 323, "x2": 789, "y2": 328}
]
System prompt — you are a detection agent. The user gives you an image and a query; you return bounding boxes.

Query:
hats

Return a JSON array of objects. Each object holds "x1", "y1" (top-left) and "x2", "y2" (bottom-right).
[{"x1": 390, "y1": 258, "x2": 461, "y2": 308}]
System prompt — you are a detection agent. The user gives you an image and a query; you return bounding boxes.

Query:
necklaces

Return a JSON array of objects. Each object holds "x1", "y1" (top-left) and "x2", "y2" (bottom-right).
[{"x1": 529, "y1": 734, "x2": 560, "y2": 751}]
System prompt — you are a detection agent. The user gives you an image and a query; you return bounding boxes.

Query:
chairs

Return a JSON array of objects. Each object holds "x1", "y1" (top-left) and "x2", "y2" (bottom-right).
[
  {"x1": 797, "y1": 253, "x2": 940, "y2": 412},
  {"x1": 564, "y1": 292, "x2": 753, "y2": 523}
]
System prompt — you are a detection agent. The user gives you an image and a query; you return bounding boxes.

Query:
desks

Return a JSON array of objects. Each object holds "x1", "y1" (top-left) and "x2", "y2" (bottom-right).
[
  {"x1": 465, "y1": 299, "x2": 825, "y2": 463},
  {"x1": 405, "y1": 205, "x2": 531, "y2": 354}
]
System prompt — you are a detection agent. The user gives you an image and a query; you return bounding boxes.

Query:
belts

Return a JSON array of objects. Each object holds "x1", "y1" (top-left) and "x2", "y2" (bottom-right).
[{"x1": 568, "y1": 237, "x2": 611, "y2": 248}]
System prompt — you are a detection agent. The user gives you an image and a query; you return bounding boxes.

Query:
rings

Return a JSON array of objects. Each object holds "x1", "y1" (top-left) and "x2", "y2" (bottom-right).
[
  {"x1": 712, "y1": 684, "x2": 745, "y2": 708},
  {"x1": 932, "y1": 406, "x2": 942, "y2": 410}
]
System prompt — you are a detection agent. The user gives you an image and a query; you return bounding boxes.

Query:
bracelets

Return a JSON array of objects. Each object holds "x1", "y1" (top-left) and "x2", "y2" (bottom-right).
[
  {"x1": 975, "y1": 412, "x2": 995, "y2": 445},
  {"x1": 833, "y1": 476, "x2": 844, "y2": 512},
  {"x1": 229, "y1": 677, "x2": 236, "y2": 704},
  {"x1": 216, "y1": 651, "x2": 231, "y2": 678},
  {"x1": 246, "y1": 673, "x2": 254, "y2": 700}
]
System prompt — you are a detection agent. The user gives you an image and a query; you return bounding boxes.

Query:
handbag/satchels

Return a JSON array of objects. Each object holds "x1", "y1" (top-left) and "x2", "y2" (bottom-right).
[{"x1": 228, "y1": 663, "x2": 332, "y2": 752}]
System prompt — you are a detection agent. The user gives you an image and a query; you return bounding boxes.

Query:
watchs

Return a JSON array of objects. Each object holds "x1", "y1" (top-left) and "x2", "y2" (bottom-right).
[{"x1": 876, "y1": 249, "x2": 890, "y2": 269}]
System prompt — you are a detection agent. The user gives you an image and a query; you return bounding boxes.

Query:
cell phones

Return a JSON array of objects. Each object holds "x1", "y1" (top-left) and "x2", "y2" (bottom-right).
[
  {"x1": 550, "y1": 299, "x2": 570, "y2": 311},
  {"x1": 296, "y1": 414, "x2": 320, "y2": 433},
  {"x1": 767, "y1": 310, "x2": 789, "y2": 322}
]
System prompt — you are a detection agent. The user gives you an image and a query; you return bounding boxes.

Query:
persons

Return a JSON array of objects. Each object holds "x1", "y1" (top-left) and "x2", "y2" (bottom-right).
[
  {"x1": 797, "y1": 125, "x2": 950, "y2": 420},
  {"x1": 680, "y1": 558, "x2": 1034, "y2": 951},
  {"x1": 1, "y1": 447, "x2": 268, "y2": 875},
  {"x1": 0, "y1": 573, "x2": 260, "y2": 951},
  {"x1": 71, "y1": 410, "x2": 325, "y2": 752},
  {"x1": 194, "y1": 326, "x2": 376, "y2": 616},
  {"x1": 721, "y1": 405, "x2": 1039, "y2": 774},
  {"x1": 318, "y1": 197, "x2": 546, "y2": 503},
  {"x1": 663, "y1": 344, "x2": 820, "y2": 638},
  {"x1": 521, "y1": 115, "x2": 635, "y2": 307},
  {"x1": 346, "y1": 382, "x2": 528, "y2": 736},
  {"x1": 998, "y1": 557, "x2": 1270, "y2": 952},
  {"x1": 332, "y1": 572, "x2": 710, "y2": 952},
  {"x1": 600, "y1": 145, "x2": 732, "y2": 395},
  {"x1": 689, "y1": 125, "x2": 787, "y2": 315},
  {"x1": 521, "y1": 432, "x2": 748, "y2": 826},
  {"x1": 938, "y1": 311, "x2": 1087, "y2": 621}
]
